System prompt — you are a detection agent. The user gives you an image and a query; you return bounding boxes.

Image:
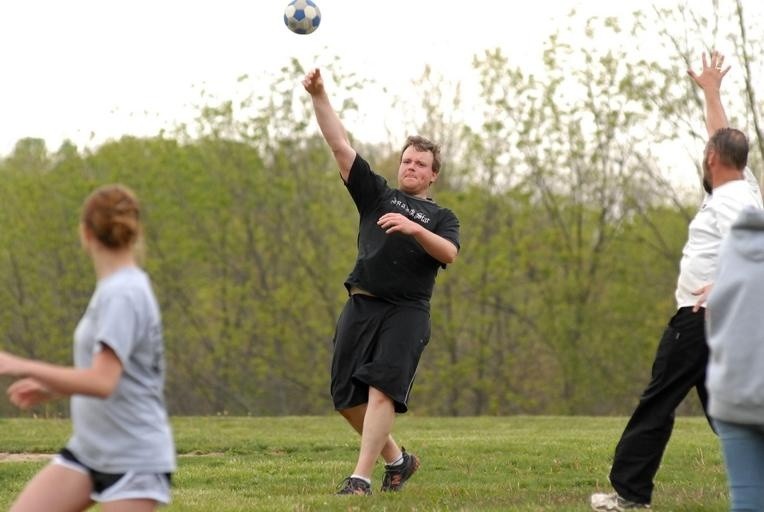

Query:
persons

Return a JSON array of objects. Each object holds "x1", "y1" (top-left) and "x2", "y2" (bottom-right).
[
  {"x1": 0, "y1": 184, "x2": 177, "y2": 512},
  {"x1": 703, "y1": 204, "x2": 764, "y2": 511},
  {"x1": 300, "y1": 67, "x2": 461, "y2": 497},
  {"x1": 590, "y1": 47, "x2": 763, "y2": 511}
]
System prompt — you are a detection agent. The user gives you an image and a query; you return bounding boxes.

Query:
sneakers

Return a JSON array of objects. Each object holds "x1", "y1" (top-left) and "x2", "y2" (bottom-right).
[
  {"x1": 334, "y1": 477, "x2": 374, "y2": 498},
  {"x1": 379, "y1": 446, "x2": 421, "y2": 492},
  {"x1": 589, "y1": 490, "x2": 652, "y2": 512}
]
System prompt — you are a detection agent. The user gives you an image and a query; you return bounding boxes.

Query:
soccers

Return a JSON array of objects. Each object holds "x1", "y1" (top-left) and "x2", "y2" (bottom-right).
[{"x1": 284, "y1": 1, "x2": 321, "y2": 34}]
